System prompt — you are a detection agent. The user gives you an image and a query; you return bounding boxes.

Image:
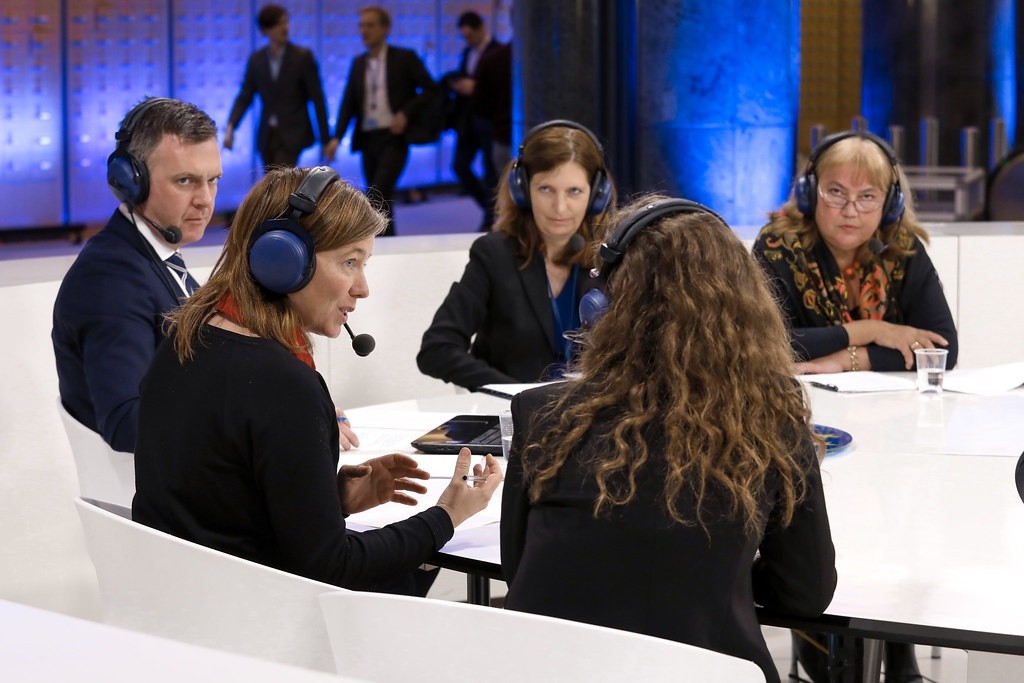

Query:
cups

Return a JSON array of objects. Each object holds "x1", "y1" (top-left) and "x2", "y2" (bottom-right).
[
  {"x1": 498, "y1": 411, "x2": 513, "y2": 461},
  {"x1": 914, "y1": 348, "x2": 948, "y2": 392}
]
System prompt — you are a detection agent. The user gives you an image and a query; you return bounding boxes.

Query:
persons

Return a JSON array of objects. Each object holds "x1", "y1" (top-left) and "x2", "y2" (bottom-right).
[
  {"x1": 453, "y1": 11, "x2": 513, "y2": 231},
  {"x1": 131, "y1": 165, "x2": 503, "y2": 598},
  {"x1": 416, "y1": 121, "x2": 617, "y2": 393},
  {"x1": 223, "y1": 6, "x2": 330, "y2": 173},
  {"x1": 751, "y1": 131, "x2": 959, "y2": 683},
  {"x1": 323, "y1": 5, "x2": 440, "y2": 237},
  {"x1": 498, "y1": 196, "x2": 837, "y2": 683},
  {"x1": 51, "y1": 95, "x2": 362, "y2": 450}
]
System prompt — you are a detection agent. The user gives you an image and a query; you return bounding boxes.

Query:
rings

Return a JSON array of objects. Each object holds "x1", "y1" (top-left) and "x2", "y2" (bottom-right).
[{"x1": 911, "y1": 341, "x2": 919, "y2": 348}]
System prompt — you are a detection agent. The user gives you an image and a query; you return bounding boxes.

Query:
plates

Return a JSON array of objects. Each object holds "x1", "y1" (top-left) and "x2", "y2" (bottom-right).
[{"x1": 813, "y1": 424, "x2": 852, "y2": 452}]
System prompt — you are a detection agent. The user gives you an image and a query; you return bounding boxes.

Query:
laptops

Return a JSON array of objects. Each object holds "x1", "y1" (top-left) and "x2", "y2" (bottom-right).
[{"x1": 411, "y1": 414, "x2": 503, "y2": 456}]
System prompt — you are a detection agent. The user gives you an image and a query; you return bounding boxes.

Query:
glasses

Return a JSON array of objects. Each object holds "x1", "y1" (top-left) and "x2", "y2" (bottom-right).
[{"x1": 818, "y1": 184, "x2": 886, "y2": 213}]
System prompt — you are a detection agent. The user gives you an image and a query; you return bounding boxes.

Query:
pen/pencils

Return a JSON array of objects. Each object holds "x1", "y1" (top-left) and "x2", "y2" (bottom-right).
[
  {"x1": 810, "y1": 381, "x2": 838, "y2": 391},
  {"x1": 463, "y1": 476, "x2": 505, "y2": 481}
]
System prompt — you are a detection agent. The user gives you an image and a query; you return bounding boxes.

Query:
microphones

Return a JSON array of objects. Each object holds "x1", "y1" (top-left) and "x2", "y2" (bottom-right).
[
  {"x1": 569, "y1": 209, "x2": 608, "y2": 252},
  {"x1": 868, "y1": 218, "x2": 901, "y2": 255},
  {"x1": 343, "y1": 323, "x2": 375, "y2": 357},
  {"x1": 127, "y1": 200, "x2": 183, "y2": 244}
]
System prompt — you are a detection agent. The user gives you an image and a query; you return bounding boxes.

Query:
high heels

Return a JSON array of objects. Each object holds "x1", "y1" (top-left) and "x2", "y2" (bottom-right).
[
  {"x1": 884, "y1": 642, "x2": 922, "y2": 683},
  {"x1": 790, "y1": 631, "x2": 829, "y2": 683}
]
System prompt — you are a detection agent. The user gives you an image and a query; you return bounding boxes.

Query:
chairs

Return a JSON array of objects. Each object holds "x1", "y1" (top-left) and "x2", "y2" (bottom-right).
[{"x1": 1, "y1": 222, "x2": 1024, "y2": 683}]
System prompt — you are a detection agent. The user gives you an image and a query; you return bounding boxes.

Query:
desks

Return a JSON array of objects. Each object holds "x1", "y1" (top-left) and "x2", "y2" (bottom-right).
[{"x1": 337, "y1": 368, "x2": 1024, "y2": 683}]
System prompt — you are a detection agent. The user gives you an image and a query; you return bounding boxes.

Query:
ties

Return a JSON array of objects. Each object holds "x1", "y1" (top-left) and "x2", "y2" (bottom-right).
[
  {"x1": 164, "y1": 252, "x2": 200, "y2": 297},
  {"x1": 371, "y1": 60, "x2": 379, "y2": 110}
]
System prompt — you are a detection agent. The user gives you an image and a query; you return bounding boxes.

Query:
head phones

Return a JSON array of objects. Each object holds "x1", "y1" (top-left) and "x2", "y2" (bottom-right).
[
  {"x1": 107, "y1": 98, "x2": 175, "y2": 214},
  {"x1": 508, "y1": 118, "x2": 613, "y2": 217},
  {"x1": 579, "y1": 197, "x2": 731, "y2": 333},
  {"x1": 792, "y1": 129, "x2": 905, "y2": 232},
  {"x1": 246, "y1": 165, "x2": 340, "y2": 297}
]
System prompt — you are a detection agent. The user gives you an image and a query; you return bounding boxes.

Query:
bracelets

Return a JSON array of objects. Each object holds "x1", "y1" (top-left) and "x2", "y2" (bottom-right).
[{"x1": 848, "y1": 346, "x2": 859, "y2": 371}]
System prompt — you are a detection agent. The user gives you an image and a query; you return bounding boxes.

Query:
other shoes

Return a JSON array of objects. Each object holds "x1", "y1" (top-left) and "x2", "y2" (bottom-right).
[{"x1": 481, "y1": 213, "x2": 493, "y2": 231}]
